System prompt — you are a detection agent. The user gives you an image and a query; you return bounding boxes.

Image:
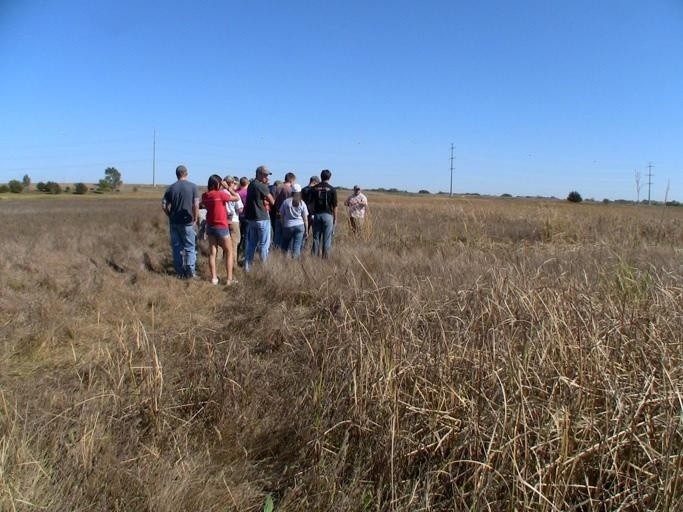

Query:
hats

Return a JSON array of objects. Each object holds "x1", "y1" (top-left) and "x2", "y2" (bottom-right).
[
  {"x1": 292, "y1": 184, "x2": 301, "y2": 193},
  {"x1": 256, "y1": 166, "x2": 272, "y2": 176},
  {"x1": 354, "y1": 185, "x2": 360, "y2": 190}
]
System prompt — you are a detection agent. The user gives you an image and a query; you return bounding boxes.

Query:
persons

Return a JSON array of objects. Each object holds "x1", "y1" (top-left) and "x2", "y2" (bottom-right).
[
  {"x1": 345, "y1": 185, "x2": 368, "y2": 234},
  {"x1": 306, "y1": 169, "x2": 338, "y2": 258},
  {"x1": 161, "y1": 166, "x2": 199, "y2": 280},
  {"x1": 301, "y1": 175, "x2": 319, "y2": 232},
  {"x1": 196, "y1": 166, "x2": 309, "y2": 285}
]
